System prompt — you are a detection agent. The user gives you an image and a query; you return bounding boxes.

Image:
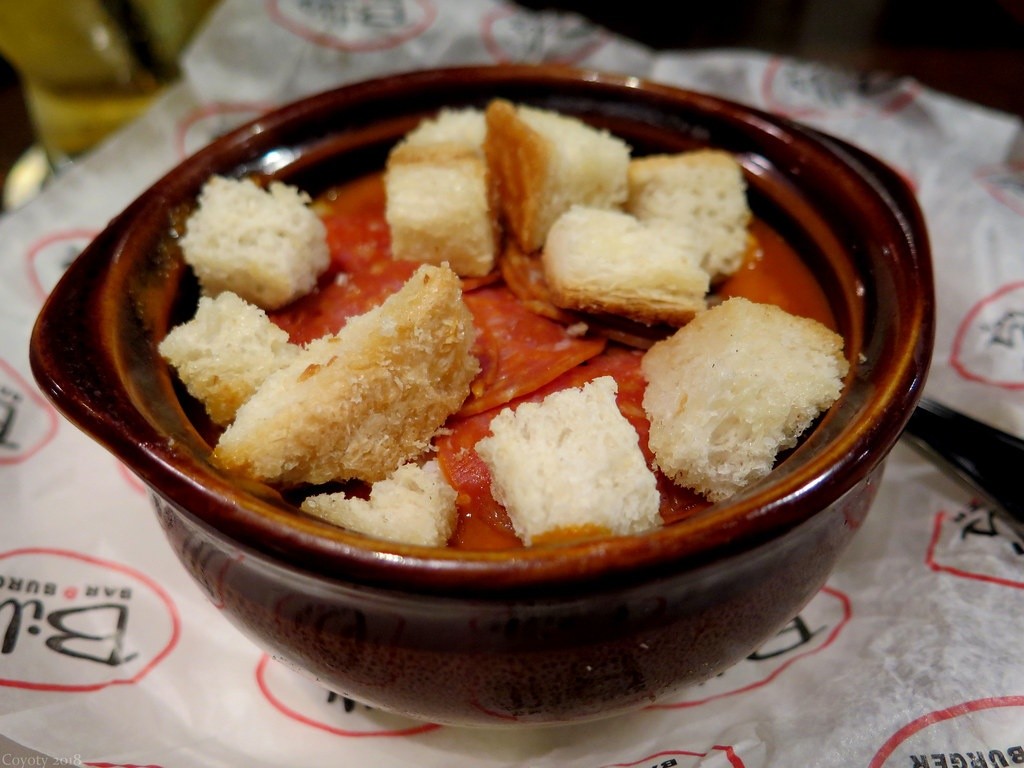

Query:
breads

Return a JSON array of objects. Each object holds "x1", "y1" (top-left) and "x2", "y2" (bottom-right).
[{"x1": 156, "y1": 102, "x2": 849, "y2": 548}]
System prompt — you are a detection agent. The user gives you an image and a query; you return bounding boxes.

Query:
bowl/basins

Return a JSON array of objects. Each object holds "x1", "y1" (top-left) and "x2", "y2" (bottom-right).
[{"x1": 26, "y1": 60, "x2": 938, "y2": 730}]
